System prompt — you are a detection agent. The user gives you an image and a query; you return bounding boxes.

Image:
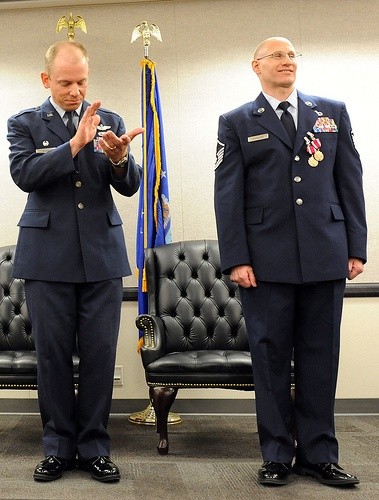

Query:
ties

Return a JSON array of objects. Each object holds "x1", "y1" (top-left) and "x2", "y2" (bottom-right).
[
  {"x1": 277, "y1": 101, "x2": 297, "y2": 147},
  {"x1": 65, "y1": 111, "x2": 77, "y2": 138}
]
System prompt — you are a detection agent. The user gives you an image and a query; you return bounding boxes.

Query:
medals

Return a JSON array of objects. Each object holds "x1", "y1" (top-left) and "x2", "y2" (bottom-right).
[{"x1": 308, "y1": 151, "x2": 324, "y2": 168}]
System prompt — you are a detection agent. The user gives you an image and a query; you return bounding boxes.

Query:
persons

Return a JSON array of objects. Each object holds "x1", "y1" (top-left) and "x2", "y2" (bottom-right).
[
  {"x1": 214, "y1": 36, "x2": 368, "y2": 486},
  {"x1": 6, "y1": 40, "x2": 146, "y2": 484}
]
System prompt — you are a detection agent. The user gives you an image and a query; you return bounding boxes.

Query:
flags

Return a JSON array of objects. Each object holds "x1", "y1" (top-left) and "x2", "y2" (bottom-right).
[{"x1": 135, "y1": 56, "x2": 172, "y2": 356}]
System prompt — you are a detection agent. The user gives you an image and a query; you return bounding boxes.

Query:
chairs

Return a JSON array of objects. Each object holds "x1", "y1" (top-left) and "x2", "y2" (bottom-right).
[
  {"x1": 0, "y1": 245, "x2": 79, "y2": 389},
  {"x1": 135, "y1": 240, "x2": 296, "y2": 455}
]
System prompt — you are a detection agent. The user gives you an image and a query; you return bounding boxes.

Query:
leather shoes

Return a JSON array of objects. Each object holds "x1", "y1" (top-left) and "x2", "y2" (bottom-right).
[
  {"x1": 78, "y1": 455, "x2": 121, "y2": 481},
  {"x1": 258, "y1": 462, "x2": 292, "y2": 484},
  {"x1": 33, "y1": 455, "x2": 76, "y2": 480},
  {"x1": 292, "y1": 456, "x2": 360, "y2": 485}
]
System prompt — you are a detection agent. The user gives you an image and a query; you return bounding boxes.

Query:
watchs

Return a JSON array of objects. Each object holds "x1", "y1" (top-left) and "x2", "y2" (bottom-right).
[{"x1": 108, "y1": 153, "x2": 128, "y2": 166}]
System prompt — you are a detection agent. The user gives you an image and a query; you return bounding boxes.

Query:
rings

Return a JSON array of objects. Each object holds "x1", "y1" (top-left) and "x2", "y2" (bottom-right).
[{"x1": 110, "y1": 146, "x2": 116, "y2": 149}]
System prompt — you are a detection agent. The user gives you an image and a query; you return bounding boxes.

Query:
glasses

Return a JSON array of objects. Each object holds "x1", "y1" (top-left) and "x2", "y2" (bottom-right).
[{"x1": 257, "y1": 51, "x2": 302, "y2": 59}]
